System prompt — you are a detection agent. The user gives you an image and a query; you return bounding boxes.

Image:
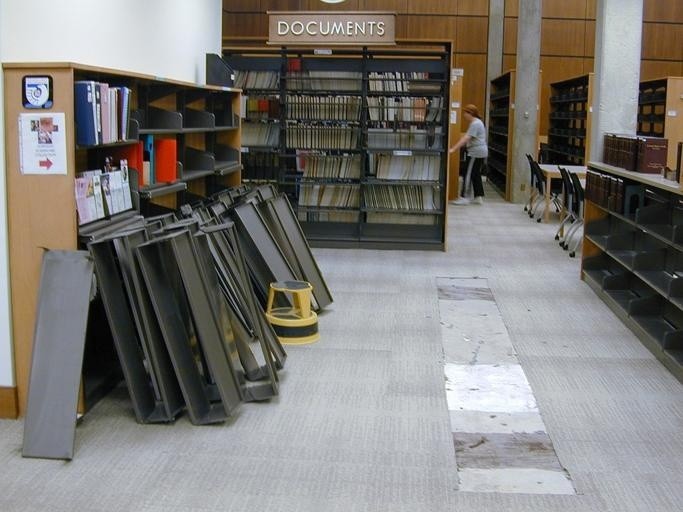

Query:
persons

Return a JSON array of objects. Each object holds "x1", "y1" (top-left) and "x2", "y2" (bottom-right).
[{"x1": 449, "y1": 104, "x2": 489, "y2": 205}]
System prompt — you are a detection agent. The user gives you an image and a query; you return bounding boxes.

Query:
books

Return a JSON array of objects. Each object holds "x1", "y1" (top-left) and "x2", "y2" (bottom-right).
[
  {"x1": 74, "y1": 80, "x2": 134, "y2": 226},
  {"x1": 586, "y1": 134, "x2": 682, "y2": 214},
  {"x1": 233, "y1": 70, "x2": 444, "y2": 225}
]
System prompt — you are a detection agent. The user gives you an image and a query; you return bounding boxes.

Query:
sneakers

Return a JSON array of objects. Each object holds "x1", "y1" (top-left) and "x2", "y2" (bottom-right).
[
  {"x1": 473, "y1": 196, "x2": 483, "y2": 204},
  {"x1": 451, "y1": 197, "x2": 471, "y2": 205}
]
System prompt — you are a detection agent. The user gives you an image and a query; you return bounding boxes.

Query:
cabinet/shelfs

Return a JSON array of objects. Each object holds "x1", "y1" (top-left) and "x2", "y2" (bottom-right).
[
  {"x1": 1, "y1": 60, "x2": 242, "y2": 425},
  {"x1": 486, "y1": 67, "x2": 682, "y2": 203},
  {"x1": 206, "y1": 34, "x2": 452, "y2": 253},
  {"x1": 579, "y1": 160, "x2": 682, "y2": 385}
]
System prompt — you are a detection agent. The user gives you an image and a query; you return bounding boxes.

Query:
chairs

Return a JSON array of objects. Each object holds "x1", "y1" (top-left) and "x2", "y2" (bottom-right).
[{"x1": 523, "y1": 151, "x2": 585, "y2": 258}]
